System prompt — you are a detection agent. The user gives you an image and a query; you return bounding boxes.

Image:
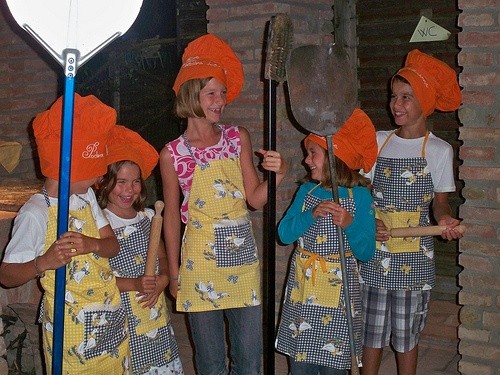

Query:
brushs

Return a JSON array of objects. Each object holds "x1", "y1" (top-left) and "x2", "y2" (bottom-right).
[{"x1": 262, "y1": 11, "x2": 296, "y2": 375}]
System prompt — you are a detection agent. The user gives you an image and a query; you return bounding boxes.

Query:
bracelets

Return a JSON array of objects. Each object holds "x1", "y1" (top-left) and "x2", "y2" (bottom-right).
[{"x1": 34, "y1": 256, "x2": 45, "y2": 278}]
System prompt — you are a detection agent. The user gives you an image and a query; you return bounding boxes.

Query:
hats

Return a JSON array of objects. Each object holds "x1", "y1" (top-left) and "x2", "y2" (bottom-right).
[
  {"x1": 172, "y1": 34, "x2": 244, "y2": 104},
  {"x1": 390, "y1": 48, "x2": 463, "y2": 118},
  {"x1": 304, "y1": 107, "x2": 379, "y2": 174},
  {"x1": 32, "y1": 91, "x2": 117, "y2": 183},
  {"x1": 107, "y1": 124, "x2": 160, "y2": 181}
]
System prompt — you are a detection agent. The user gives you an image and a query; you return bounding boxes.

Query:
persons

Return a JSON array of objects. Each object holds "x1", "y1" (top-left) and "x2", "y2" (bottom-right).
[
  {"x1": 0, "y1": 95, "x2": 184, "y2": 375},
  {"x1": 276, "y1": 109, "x2": 379, "y2": 375},
  {"x1": 354, "y1": 46, "x2": 465, "y2": 375},
  {"x1": 157, "y1": 34, "x2": 287, "y2": 374}
]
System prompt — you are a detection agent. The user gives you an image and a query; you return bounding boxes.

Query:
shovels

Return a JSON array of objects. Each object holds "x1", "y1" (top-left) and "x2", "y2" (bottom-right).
[
  {"x1": 5, "y1": 0, "x2": 146, "y2": 375},
  {"x1": 283, "y1": 43, "x2": 360, "y2": 375}
]
